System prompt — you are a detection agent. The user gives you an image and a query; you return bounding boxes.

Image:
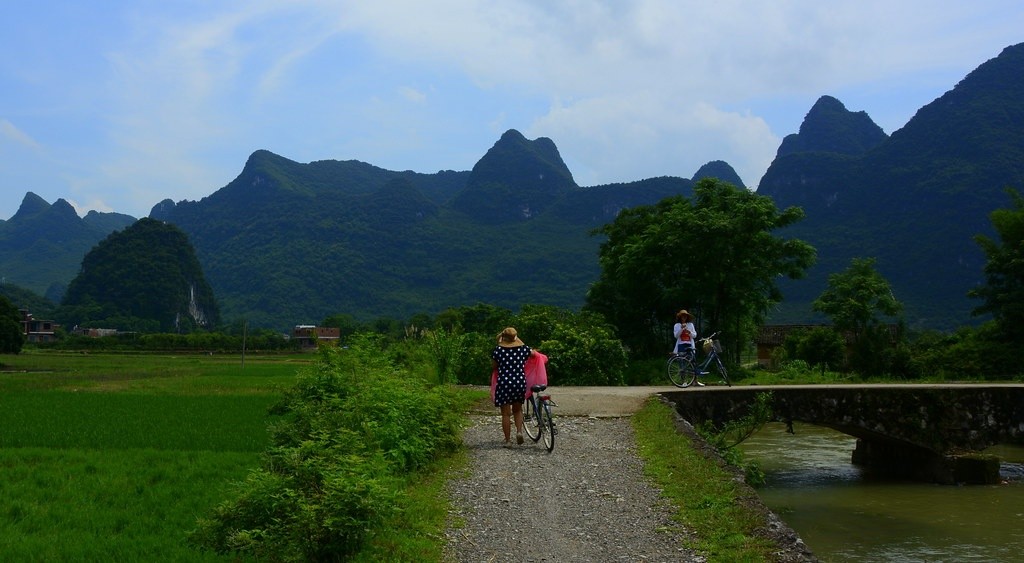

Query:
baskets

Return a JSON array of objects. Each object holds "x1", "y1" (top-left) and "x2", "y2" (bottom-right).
[{"x1": 707, "y1": 339, "x2": 722, "y2": 354}]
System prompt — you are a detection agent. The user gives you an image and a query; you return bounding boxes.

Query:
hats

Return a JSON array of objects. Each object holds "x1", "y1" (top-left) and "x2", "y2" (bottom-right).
[
  {"x1": 496, "y1": 327, "x2": 524, "y2": 347},
  {"x1": 676, "y1": 310, "x2": 696, "y2": 323}
]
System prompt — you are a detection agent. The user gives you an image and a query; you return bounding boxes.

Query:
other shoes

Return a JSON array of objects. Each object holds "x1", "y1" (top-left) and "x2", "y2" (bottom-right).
[
  {"x1": 517, "y1": 433, "x2": 524, "y2": 445},
  {"x1": 683, "y1": 381, "x2": 688, "y2": 386},
  {"x1": 692, "y1": 382, "x2": 705, "y2": 386},
  {"x1": 503, "y1": 439, "x2": 512, "y2": 448}
]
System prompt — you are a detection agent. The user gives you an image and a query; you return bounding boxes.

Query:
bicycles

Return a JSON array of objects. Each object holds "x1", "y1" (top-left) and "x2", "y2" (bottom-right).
[
  {"x1": 522, "y1": 383, "x2": 559, "y2": 452},
  {"x1": 666, "y1": 331, "x2": 731, "y2": 388}
]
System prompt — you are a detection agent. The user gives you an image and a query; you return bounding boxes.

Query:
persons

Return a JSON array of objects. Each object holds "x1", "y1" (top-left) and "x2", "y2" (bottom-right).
[
  {"x1": 491, "y1": 327, "x2": 548, "y2": 448},
  {"x1": 673, "y1": 310, "x2": 704, "y2": 387}
]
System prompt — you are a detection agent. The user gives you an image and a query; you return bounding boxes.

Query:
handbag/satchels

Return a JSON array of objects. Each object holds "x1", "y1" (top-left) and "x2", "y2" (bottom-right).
[{"x1": 678, "y1": 343, "x2": 692, "y2": 352}]
